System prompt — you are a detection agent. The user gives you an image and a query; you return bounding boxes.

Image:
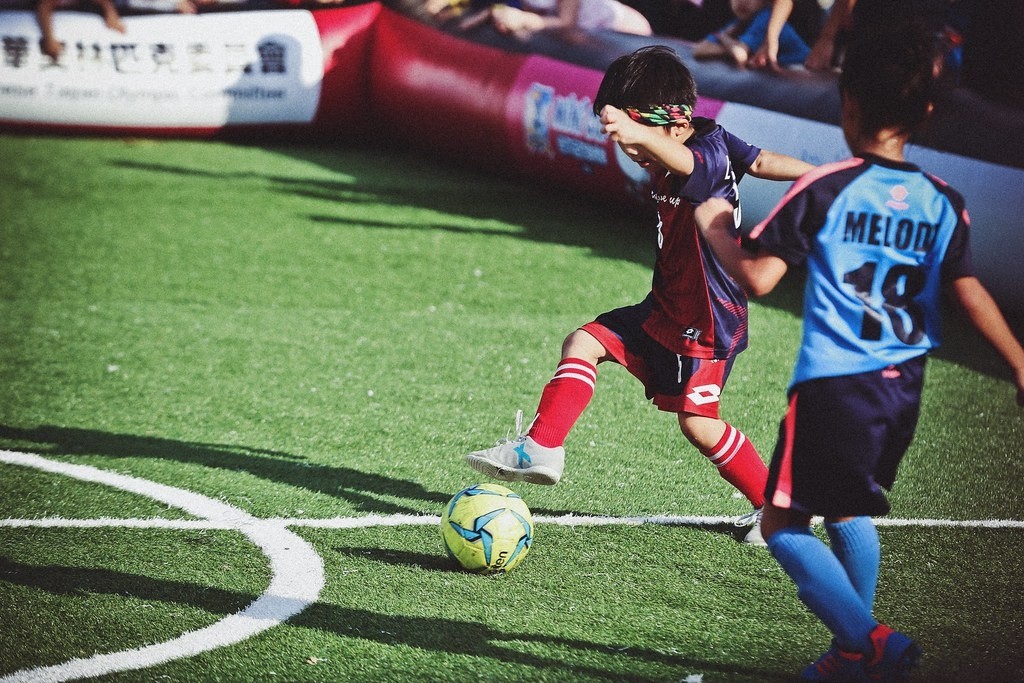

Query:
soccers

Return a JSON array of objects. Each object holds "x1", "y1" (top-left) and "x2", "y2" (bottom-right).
[{"x1": 441, "y1": 484, "x2": 534, "y2": 573}]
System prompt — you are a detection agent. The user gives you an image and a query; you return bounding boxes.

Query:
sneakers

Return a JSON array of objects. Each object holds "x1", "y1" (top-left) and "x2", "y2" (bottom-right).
[
  {"x1": 734, "y1": 505, "x2": 768, "y2": 546},
  {"x1": 801, "y1": 637, "x2": 863, "y2": 682},
  {"x1": 467, "y1": 436, "x2": 566, "y2": 485},
  {"x1": 863, "y1": 625, "x2": 919, "y2": 683}
]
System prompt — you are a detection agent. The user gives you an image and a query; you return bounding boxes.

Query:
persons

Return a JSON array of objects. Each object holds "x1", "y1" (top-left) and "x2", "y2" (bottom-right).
[
  {"x1": 466, "y1": 44, "x2": 826, "y2": 547},
  {"x1": 693, "y1": 17, "x2": 1024, "y2": 683},
  {"x1": 35, "y1": 0, "x2": 1023, "y2": 119}
]
